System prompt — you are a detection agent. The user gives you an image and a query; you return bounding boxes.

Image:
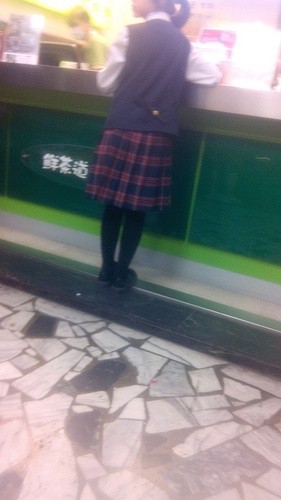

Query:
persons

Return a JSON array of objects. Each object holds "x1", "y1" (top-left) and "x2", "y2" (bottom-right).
[{"x1": 85, "y1": 0, "x2": 222, "y2": 292}]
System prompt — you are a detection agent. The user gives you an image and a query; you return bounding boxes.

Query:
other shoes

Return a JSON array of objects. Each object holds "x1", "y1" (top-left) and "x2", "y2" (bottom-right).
[{"x1": 96, "y1": 268, "x2": 138, "y2": 292}]
